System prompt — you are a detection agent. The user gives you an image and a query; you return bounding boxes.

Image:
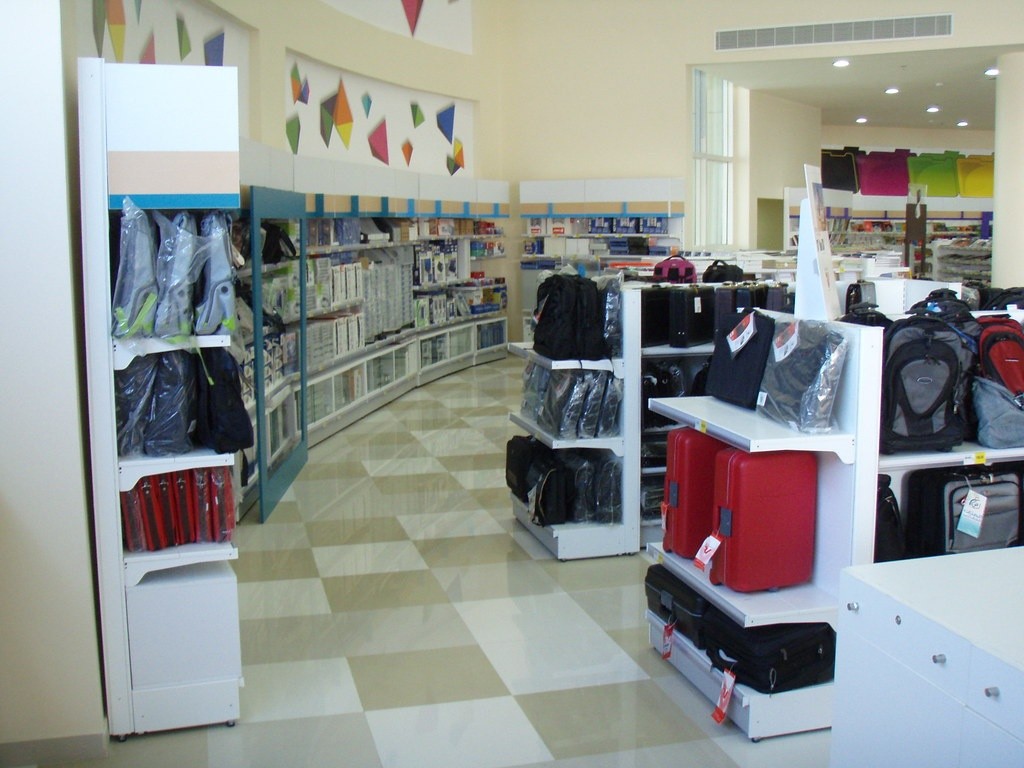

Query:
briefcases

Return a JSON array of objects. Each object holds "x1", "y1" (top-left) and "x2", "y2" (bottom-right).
[
  {"x1": 703, "y1": 609, "x2": 836, "y2": 694},
  {"x1": 645, "y1": 564, "x2": 712, "y2": 648},
  {"x1": 639, "y1": 281, "x2": 797, "y2": 348}
]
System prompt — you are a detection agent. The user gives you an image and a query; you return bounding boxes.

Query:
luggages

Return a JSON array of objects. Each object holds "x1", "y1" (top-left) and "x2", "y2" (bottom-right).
[
  {"x1": 662, "y1": 427, "x2": 731, "y2": 559},
  {"x1": 710, "y1": 446, "x2": 818, "y2": 593}
]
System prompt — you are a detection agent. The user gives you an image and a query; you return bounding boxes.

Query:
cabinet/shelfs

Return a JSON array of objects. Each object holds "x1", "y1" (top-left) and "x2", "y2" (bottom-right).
[{"x1": 75, "y1": 57, "x2": 1024, "y2": 739}]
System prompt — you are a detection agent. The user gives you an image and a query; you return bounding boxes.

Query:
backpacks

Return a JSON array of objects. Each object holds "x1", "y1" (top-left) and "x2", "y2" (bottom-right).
[
  {"x1": 969, "y1": 313, "x2": 1024, "y2": 448},
  {"x1": 195, "y1": 347, "x2": 254, "y2": 453},
  {"x1": 702, "y1": 261, "x2": 743, "y2": 282},
  {"x1": 880, "y1": 311, "x2": 977, "y2": 454},
  {"x1": 145, "y1": 350, "x2": 197, "y2": 454},
  {"x1": 533, "y1": 275, "x2": 606, "y2": 361},
  {"x1": 906, "y1": 288, "x2": 974, "y2": 324},
  {"x1": 110, "y1": 212, "x2": 156, "y2": 338},
  {"x1": 654, "y1": 255, "x2": 696, "y2": 285},
  {"x1": 154, "y1": 211, "x2": 197, "y2": 343},
  {"x1": 116, "y1": 356, "x2": 159, "y2": 454},
  {"x1": 193, "y1": 212, "x2": 239, "y2": 335},
  {"x1": 839, "y1": 302, "x2": 895, "y2": 328}
]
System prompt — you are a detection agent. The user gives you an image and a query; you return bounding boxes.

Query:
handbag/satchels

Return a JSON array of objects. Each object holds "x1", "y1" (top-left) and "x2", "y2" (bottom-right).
[
  {"x1": 707, "y1": 307, "x2": 847, "y2": 434},
  {"x1": 119, "y1": 466, "x2": 234, "y2": 552},
  {"x1": 873, "y1": 474, "x2": 908, "y2": 563},
  {"x1": 523, "y1": 365, "x2": 621, "y2": 440},
  {"x1": 847, "y1": 279, "x2": 877, "y2": 309},
  {"x1": 906, "y1": 460, "x2": 1024, "y2": 558},
  {"x1": 503, "y1": 436, "x2": 622, "y2": 526}
]
